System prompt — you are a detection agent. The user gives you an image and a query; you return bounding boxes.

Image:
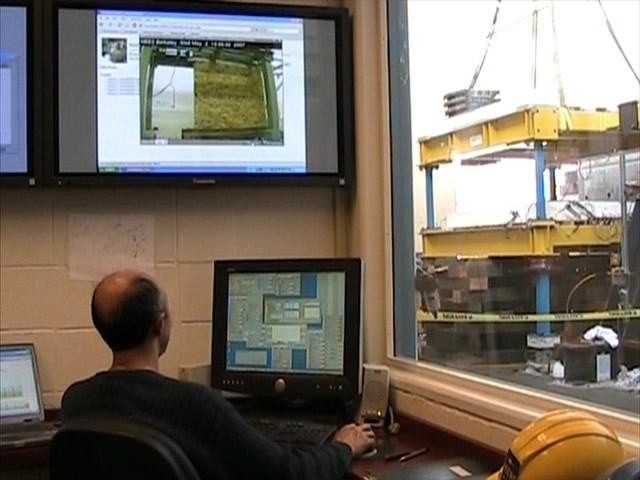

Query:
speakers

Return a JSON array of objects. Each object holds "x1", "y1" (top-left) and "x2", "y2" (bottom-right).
[
  {"x1": 178, "y1": 363, "x2": 222, "y2": 397},
  {"x1": 358, "y1": 364, "x2": 391, "y2": 428}
]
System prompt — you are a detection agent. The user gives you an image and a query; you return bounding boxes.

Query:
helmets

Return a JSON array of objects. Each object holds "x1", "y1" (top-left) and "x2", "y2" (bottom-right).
[{"x1": 486, "y1": 408, "x2": 625, "y2": 480}]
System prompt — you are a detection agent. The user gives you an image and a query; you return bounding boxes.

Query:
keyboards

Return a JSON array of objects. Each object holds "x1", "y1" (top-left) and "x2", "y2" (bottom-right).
[{"x1": 243, "y1": 417, "x2": 338, "y2": 447}]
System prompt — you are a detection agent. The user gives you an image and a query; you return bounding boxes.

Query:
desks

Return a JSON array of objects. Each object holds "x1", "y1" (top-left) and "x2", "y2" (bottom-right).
[{"x1": 0, "y1": 403, "x2": 508, "y2": 480}]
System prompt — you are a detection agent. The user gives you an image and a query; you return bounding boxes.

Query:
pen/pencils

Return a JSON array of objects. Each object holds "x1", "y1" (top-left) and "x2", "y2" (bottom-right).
[
  {"x1": 400, "y1": 446, "x2": 429, "y2": 462},
  {"x1": 384, "y1": 452, "x2": 409, "y2": 461}
]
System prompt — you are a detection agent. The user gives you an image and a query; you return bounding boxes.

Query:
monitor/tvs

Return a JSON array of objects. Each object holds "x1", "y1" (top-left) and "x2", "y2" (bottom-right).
[
  {"x1": 211, "y1": 256, "x2": 364, "y2": 398},
  {"x1": 0, "y1": 0, "x2": 42, "y2": 189},
  {"x1": 42, "y1": 0, "x2": 354, "y2": 190}
]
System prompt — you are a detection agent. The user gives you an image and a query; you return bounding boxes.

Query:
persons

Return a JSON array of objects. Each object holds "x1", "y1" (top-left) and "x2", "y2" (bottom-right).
[{"x1": 60, "y1": 270, "x2": 376, "y2": 480}]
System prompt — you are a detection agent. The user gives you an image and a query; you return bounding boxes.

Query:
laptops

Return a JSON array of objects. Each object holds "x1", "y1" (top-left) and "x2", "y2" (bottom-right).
[{"x1": 0, "y1": 342, "x2": 57, "y2": 448}]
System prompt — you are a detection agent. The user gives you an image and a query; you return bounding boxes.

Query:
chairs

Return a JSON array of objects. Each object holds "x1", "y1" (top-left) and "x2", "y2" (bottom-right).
[{"x1": 38, "y1": 419, "x2": 194, "y2": 479}]
